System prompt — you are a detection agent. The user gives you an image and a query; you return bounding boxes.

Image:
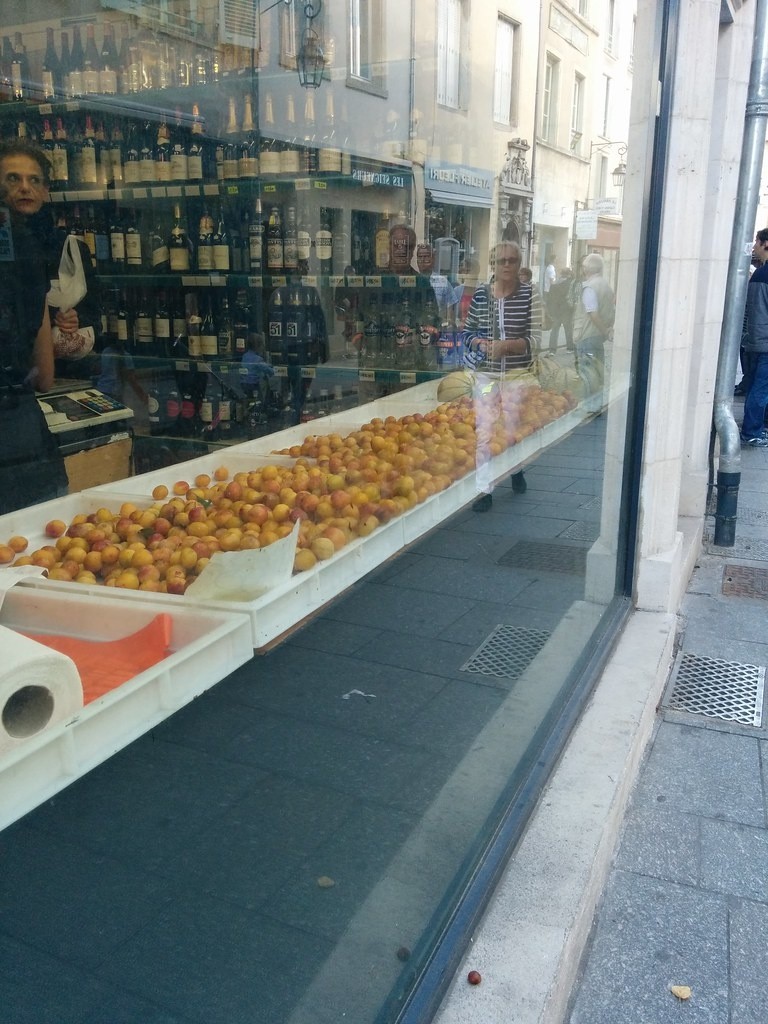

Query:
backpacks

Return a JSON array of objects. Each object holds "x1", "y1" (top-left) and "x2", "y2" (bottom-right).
[{"x1": 549, "y1": 284, "x2": 567, "y2": 324}]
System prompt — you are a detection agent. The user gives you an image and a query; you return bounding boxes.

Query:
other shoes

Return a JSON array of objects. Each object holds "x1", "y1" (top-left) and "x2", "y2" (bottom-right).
[
  {"x1": 734, "y1": 385, "x2": 745, "y2": 396},
  {"x1": 474, "y1": 493, "x2": 493, "y2": 510},
  {"x1": 566, "y1": 349, "x2": 574, "y2": 354},
  {"x1": 740, "y1": 431, "x2": 768, "y2": 446},
  {"x1": 511, "y1": 472, "x2": 527, "y2": 493},
  {"x1": 549, "y1": 350, "x2": 555, "y2": 356}
]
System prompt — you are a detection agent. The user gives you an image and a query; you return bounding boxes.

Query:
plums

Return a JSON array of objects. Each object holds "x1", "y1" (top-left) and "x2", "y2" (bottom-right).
[{"x1": 0, "y1": 386, "x2": 576, "y2": 596}]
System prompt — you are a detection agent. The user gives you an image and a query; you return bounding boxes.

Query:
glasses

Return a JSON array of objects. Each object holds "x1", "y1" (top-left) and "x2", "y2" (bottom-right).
[{"x1": 497, "y1": 256, "x2": 520, "y2": 266}]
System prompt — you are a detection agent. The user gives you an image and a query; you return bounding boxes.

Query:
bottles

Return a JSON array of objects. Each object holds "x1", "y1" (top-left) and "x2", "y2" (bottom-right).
[{"x1": 1, "y1": 0, "x2": 469, "y2": 443}]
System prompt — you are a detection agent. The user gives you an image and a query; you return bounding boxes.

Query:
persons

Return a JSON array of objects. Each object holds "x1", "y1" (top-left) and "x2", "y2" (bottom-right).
[
  {"x1": 221, "y1": 225, "x2": 615, "y2": 510},
  {"x1": 0, "y1": 138, "x2": 145, "y2": 518},
  {"x1": 733, "y1": 228, "x2": 768, "y2": 448}
]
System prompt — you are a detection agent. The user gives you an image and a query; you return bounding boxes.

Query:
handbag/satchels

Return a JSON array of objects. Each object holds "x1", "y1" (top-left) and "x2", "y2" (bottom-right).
[{"x1": 46, "y1": 234, "x2": 97, "y2": 361}]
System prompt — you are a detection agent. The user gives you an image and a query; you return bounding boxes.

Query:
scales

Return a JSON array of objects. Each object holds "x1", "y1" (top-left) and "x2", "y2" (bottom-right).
[{"x1": 35, "y1": 378, "x2": 134, "y2": 458}]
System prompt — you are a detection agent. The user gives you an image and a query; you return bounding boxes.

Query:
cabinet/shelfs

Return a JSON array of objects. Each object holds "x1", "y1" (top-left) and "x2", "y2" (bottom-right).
[{"x1": 0, "y1": 56, "x2": 469, "y2": 475}]
